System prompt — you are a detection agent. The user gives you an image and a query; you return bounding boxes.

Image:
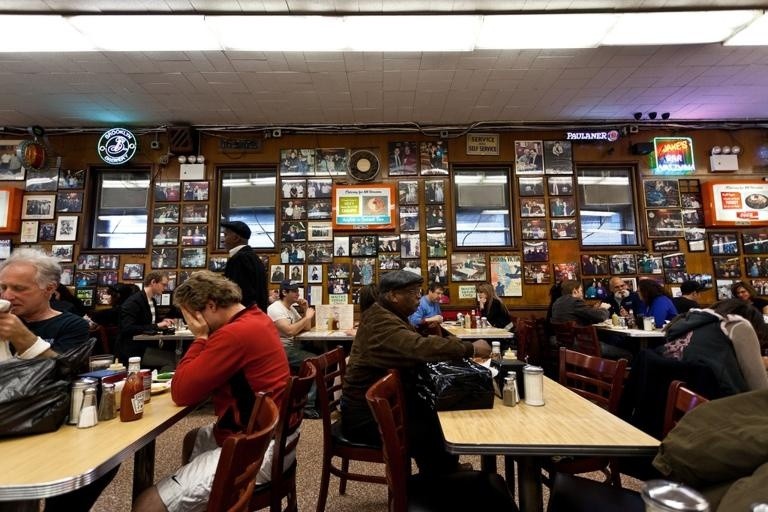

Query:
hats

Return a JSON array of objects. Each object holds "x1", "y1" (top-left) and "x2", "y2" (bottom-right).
[
  {"x1": 220, "y1": 221, "x2": 250, "y2": 240},
  {"x1": 380, "y1": 271, "x2": 422, "y2": 289},
  {"x1": 280, "y1": 279, "x2": 304, "y2": 290}
]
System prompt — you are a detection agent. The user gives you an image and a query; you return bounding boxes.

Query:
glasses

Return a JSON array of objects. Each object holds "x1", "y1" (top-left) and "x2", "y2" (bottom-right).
[{"x1": 159, "y1": 282, "x2": 169, "y2": 286}]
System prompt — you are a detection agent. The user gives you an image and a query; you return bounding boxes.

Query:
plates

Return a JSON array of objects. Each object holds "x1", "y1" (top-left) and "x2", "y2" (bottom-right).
[{"x1": 151, "y1": 383, "x2": 169, "y2": 395}]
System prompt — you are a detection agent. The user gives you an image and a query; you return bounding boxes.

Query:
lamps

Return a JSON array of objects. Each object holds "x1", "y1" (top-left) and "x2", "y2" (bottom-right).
[
  {"x1": 648, "y1": 112, "x2": 657, "y2": 119},
  {"x1": 661, "y1": 112, "x2": 670, "y2": 120},
  {"x1": 633, "y1": 111, "x2": 642, "y2": 120}
]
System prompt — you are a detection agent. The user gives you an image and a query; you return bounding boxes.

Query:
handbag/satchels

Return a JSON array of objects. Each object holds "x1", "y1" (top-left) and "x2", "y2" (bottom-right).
[
  {"x1": 418, "y1": 356, "x2": 498, "y2": 411},
  {"x1": 1, "y1": 337, "x2": 96, "y2": 440}
]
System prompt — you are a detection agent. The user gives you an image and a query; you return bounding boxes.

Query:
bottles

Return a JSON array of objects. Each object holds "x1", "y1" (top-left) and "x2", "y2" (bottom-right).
[
  {"x1": 642, "y1": 479, "x2": 707, "y2": 509},
  {"x1": 491, "y1": 342, "x2": 502, "y2": 361},
  {"x1": 503, "y1": 366, "x2": 546, "y2": 407},
  {"x1": 327, "y1": 312, "x2": 340, "y2": 329},
  {"x1": 107, "y1": 358, "x2": 126, "y2": 411},
  {"x1": 457, "y1": 308, "x2": 487, "y2": 329},
  {"x1": 607, "y1": 309, "x2": 655, "y2": 330},
  {"x1": 120, "y1": 355, "x2": 145, "y2": 421},
  {"x1": 67, "y1": 377, "x2": 116, "y2": 430},
  {"x1": 503, "y1": 347, "x2": 517, "y2": 360}
]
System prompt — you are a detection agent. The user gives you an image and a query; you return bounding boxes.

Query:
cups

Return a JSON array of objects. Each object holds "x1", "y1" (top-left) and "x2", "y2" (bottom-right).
[
  {"x1": 174, "y1": 319, "x2": 185, "y2": 331},
  {"x1": 88, "y1": 354, "x2": 114, "y2": 373}
]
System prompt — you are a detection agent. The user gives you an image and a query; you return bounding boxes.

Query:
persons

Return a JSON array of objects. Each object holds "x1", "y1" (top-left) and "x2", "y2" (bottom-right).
[
  {"x1": 407, "y1": 282, "x2": 447, "y2": 338},
  {"x1": 351, "y1": 140, "x2": 447, "y2": 286},
  {"x1": 265, "y1": 279, "x2": 322, "y2": 419},
  {"x1": 342, "y1": 271, "x2": 491, "y2": 473},
  {"x1": 0, "y1": 248, "x2": 122, "y2": 512},
  {"x1": 131, "y1": 270, "x2": 301, "y2": 511},
  {"x1": 343, "y1": 284, "x2": 378, "y2": 340},
  {"x1": 710, "y1": 232, "x2": 768, "y2": 300},
  {"x1": 478, "y1": 283, "x2": 516, "y2": 353},
  {"x1": 219, "y1": 221, "x2": 267, "y2": 312},
  {"x1": 270, "y1": 151, "x2": 345, "y2": 298},
  {"x1": 541, "y1": 269, "x2": 767, "y2": 509},
  {"x1": 48, "y1": 263, "x2": 86, "y2": 318},
  {"x1": 516, "y1": 141, "x2": 581, "y2": 284},
  {"x1": 581, "y1": 252, "x2": 712, "y2": 299},
  {"x1": 454, "y1": 253, "x2": 522, "y2": 296},
  {"x1": 0, "y1": 146, "x2": 209, "y2": 306},
  {"x1": 113, "y1": 270, "x2": 178, "y2": 374},
  {"x1": 646, "y1": 178, "x2": 705, "y2": 251}
]
